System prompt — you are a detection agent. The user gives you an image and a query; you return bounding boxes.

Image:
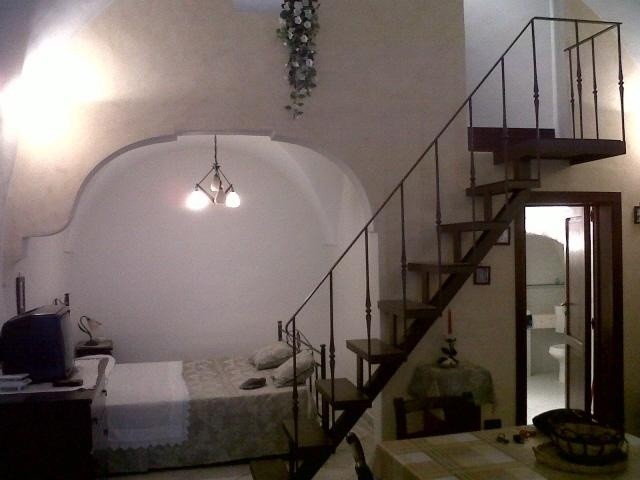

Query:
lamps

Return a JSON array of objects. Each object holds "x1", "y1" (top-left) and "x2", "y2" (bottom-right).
[
  {"x1": 78, "y1": 315, "x2": 103, "y2": 347},
  {"x1": 184, "y1": 134, "x2": 240, "y2": 210}
]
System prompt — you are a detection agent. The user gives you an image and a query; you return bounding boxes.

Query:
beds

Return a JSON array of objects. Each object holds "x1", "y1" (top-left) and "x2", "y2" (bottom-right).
[{"x1": 75, "y1": 320, "x2": 327, "y2": 477}]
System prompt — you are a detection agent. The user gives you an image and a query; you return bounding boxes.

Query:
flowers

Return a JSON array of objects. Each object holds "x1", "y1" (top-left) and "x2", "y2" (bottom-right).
[{"x1": 275, "y1": 0, "x2": 321, "y2": 120}]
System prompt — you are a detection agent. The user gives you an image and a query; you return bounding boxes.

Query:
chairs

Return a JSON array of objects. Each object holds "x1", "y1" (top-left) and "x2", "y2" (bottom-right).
[{"x1": 346, "y1": 391, "x2": 482, "y2": 480}]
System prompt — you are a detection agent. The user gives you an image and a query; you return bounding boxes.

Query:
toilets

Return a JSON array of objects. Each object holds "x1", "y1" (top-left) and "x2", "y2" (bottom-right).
[{"x1": 549, "y1": 344, "x2": 565, "y2": 381}]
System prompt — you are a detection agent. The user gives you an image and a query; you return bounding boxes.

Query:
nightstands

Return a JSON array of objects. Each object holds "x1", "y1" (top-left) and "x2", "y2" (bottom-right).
[{"x1": 76, "y1": 340, "x2": 113, "y2": 357}]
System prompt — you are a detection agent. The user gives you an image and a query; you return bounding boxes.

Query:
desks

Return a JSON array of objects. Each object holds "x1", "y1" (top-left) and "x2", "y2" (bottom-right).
[
  {"x1": 370, "y1": 425, "x2": 640, "y2": 480},
  {"x1": 408, "y1": 359, "x2": 497, "y2": 433}
]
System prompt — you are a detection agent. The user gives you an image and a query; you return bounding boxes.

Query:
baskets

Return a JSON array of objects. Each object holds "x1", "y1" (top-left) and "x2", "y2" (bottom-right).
[
  {"x1": 532, "y1": 440, "x2": 628, "y2": 473},
  {"x1": 551, "y1": 421, "x2": 625, "y2": 462}
]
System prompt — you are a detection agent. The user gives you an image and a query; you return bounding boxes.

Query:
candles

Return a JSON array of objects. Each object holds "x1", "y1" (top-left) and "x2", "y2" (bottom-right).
[{"x1": 447, "y1": 309, "x2": 452, "y2": 335}]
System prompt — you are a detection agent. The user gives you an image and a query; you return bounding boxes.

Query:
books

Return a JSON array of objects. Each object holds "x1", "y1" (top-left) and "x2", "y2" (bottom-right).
[
  {"x1": 0, "y1": 373, "x2": 30, "y2": 381},
  {"x1": 0, "y1": 378, "x2": 33, "y2": 392}
]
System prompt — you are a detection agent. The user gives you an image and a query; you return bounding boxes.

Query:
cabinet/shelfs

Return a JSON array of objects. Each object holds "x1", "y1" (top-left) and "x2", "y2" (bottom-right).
[{"x1": 0, "y1": 353, "x2": 112, "y2": 480}]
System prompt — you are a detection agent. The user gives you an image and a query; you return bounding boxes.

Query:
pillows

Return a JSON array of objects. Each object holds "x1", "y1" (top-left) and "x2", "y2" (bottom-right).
[{"x1": 248, "y1": 340, "x2": 316, "y2": 388}]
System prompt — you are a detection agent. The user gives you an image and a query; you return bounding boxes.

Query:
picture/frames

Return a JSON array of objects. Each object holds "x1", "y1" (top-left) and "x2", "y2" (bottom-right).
[
  {"x1": 494, "y1": 226, "x2": 511, "y2": 245},
  {"x1": 473, "y1": 266, "x2": 490, "y2": 284}
]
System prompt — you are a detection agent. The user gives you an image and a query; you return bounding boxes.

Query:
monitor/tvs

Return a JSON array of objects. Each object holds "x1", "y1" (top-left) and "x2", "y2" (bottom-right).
[{"x1": 0, "y1": 304, "x2": 76, "y2": 384}]
